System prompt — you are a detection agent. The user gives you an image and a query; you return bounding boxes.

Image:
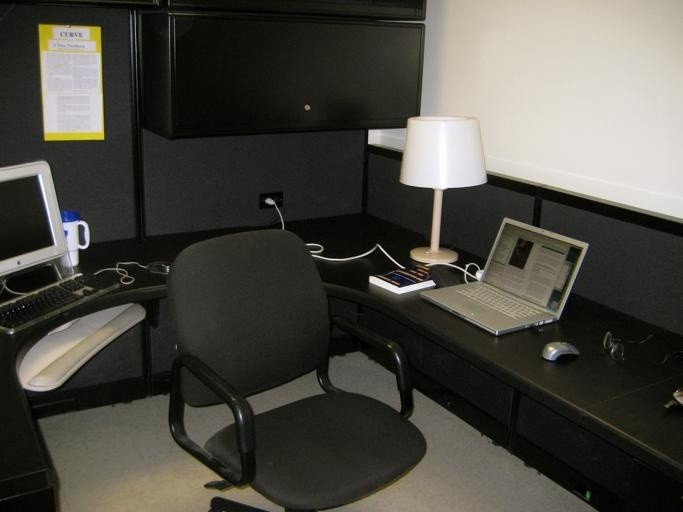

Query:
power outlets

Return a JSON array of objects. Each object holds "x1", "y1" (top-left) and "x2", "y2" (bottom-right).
[{"x1": 259, "y1": 192, "x2": 283, "y2": 208}]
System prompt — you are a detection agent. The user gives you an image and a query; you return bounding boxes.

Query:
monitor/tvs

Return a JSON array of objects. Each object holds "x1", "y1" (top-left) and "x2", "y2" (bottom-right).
[{"x1": 0, "y1": 160, "x2": 75, "y2": 281}]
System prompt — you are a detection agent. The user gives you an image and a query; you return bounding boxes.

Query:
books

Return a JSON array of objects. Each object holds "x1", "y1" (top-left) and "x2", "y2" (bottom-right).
[{"x1": 369, "y1": 264, "x2": 436, "y2": 295}]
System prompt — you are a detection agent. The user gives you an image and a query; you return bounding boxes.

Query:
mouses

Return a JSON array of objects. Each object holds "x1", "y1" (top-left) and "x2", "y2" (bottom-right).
[{"x1": 541, "y1": 341, "x2": 581, "y2": 362}]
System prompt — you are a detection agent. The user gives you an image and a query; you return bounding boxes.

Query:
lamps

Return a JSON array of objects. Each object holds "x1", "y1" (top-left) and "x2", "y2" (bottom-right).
[{"x1": 398, "y1": 116, "x2": 487, "y2": 265}]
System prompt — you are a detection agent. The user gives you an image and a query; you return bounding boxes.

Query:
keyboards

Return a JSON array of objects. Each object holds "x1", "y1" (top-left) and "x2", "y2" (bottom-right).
[{"x1": 0, "y1": 272, "x2": 121, "y2": 335}]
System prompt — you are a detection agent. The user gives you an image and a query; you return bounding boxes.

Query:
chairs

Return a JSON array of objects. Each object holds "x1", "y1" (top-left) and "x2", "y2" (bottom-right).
[{"x1": 166, "y1": 229, "x2": 427, "y2": 511}]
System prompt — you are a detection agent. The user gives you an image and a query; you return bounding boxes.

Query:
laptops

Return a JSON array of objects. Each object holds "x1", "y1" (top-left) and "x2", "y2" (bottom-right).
[{"x1": 419, "y1": 216, "x2": 590, "y2": 336}]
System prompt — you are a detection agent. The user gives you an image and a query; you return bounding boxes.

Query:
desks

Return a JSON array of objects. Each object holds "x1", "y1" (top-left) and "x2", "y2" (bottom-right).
[{"x1": 2, "y1": 211, "x2": 683, "y2": 512}]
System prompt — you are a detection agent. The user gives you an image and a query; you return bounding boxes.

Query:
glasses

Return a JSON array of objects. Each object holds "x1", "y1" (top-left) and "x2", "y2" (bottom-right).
[{"x1": 603, "y1": 329, "x2": 672, "y2": 362}]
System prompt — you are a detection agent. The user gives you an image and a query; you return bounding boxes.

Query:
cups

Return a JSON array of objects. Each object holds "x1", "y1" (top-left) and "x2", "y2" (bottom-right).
[{"x1": 57, "y1": 209, "x2": 90, "y2": 268}]
